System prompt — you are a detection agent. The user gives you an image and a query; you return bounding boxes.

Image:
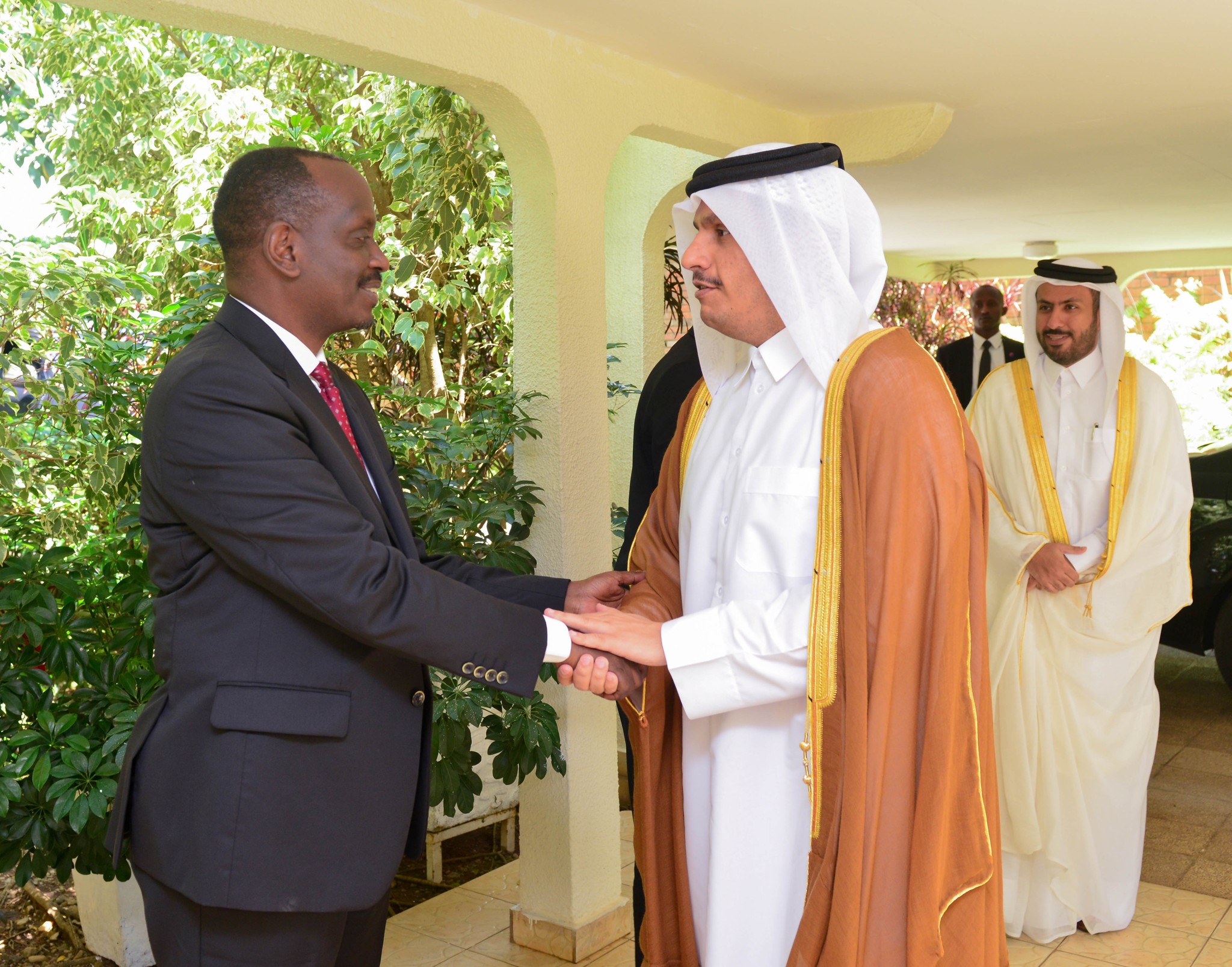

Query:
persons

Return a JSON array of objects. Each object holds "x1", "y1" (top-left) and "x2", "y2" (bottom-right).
[
  {"x1": 613, "y1": 325, "x2": 701, "y2": 967},
  {"x1": 937, "y1": 285, "x2": 1025, "y2": 411},
  {"x1": 543, "y1": 141, "x2": 1008, "y2": 967},
  {"x1": 103, "y1": 145, "x2": 642, "y2": 967},
  {"x1": 963, "y1": 256, "x2": 1195, "y2": 945}
]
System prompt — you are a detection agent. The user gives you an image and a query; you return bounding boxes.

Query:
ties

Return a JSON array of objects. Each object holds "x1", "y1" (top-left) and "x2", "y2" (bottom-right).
[
  {"x1": 977, "y1": 341, "x2": 992, "y2": 389},
  {"x1": 313, "y1": 362, "x2": 365, "y2": 470}
]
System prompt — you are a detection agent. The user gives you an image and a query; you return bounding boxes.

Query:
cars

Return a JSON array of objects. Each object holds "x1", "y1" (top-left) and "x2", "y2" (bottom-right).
[{"x1": 1157, "y1": 444, "x2": 1231, "y2": 686}]
